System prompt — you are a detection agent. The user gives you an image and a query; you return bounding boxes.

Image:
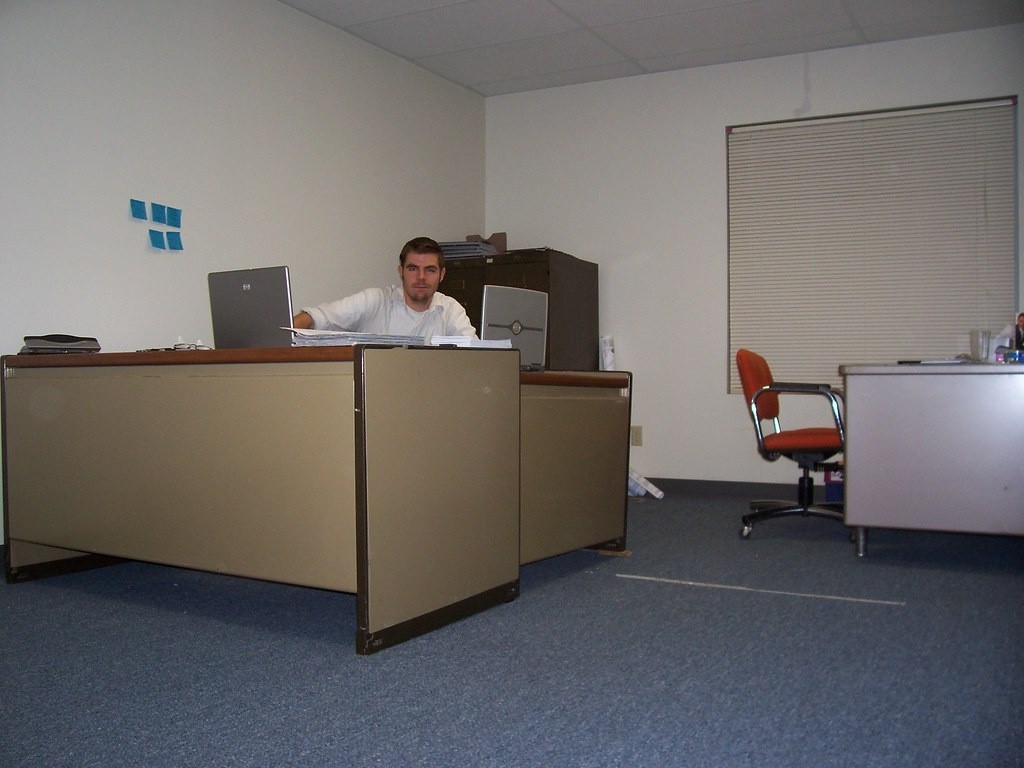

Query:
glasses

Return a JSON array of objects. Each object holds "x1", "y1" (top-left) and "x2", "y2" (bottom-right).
[{"x1": 174, "y1": 343, "x2": 212, "y2": 350}]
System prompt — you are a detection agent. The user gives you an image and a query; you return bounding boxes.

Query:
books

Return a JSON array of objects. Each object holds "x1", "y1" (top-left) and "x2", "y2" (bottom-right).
[{"x1": 438, "y1": 241, "x2": 499, "y2": 260}]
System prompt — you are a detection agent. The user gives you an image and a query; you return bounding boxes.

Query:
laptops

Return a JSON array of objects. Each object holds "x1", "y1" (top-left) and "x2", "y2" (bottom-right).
[
  {"x1": 480, "y1": 285, "x2": 549, "y2": 372},
  {"x1": 208, "y1": 266, "x2": 297, "y2": 348}
]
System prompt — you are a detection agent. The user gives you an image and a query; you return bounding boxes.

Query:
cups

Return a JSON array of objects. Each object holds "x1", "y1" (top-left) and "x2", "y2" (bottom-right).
[{"x1": 976, "y1": 329, "x2": 991, "y2": 362}]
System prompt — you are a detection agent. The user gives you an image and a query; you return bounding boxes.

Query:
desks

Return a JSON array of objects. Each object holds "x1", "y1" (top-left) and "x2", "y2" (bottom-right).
[
  {"x1": 839, "y1": 362, "x2": 1024, "y2": 557},
  {"x1": 0, "y1": 342, "x2": 633, "y2": 654}
]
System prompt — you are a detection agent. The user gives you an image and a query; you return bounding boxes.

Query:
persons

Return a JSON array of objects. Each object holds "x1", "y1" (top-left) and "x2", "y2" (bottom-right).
[
  {"x1": 1016, "y1": 312, "x2": 1024, "y2": 350},
  {"x1": 293, "y1": 236, "x2": 479, "y2": 340}
]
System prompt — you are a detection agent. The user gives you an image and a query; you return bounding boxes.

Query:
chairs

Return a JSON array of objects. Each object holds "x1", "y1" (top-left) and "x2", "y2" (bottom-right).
[{"x1": 736, "y1": 348, "x2": 855, "y2": 544}]
